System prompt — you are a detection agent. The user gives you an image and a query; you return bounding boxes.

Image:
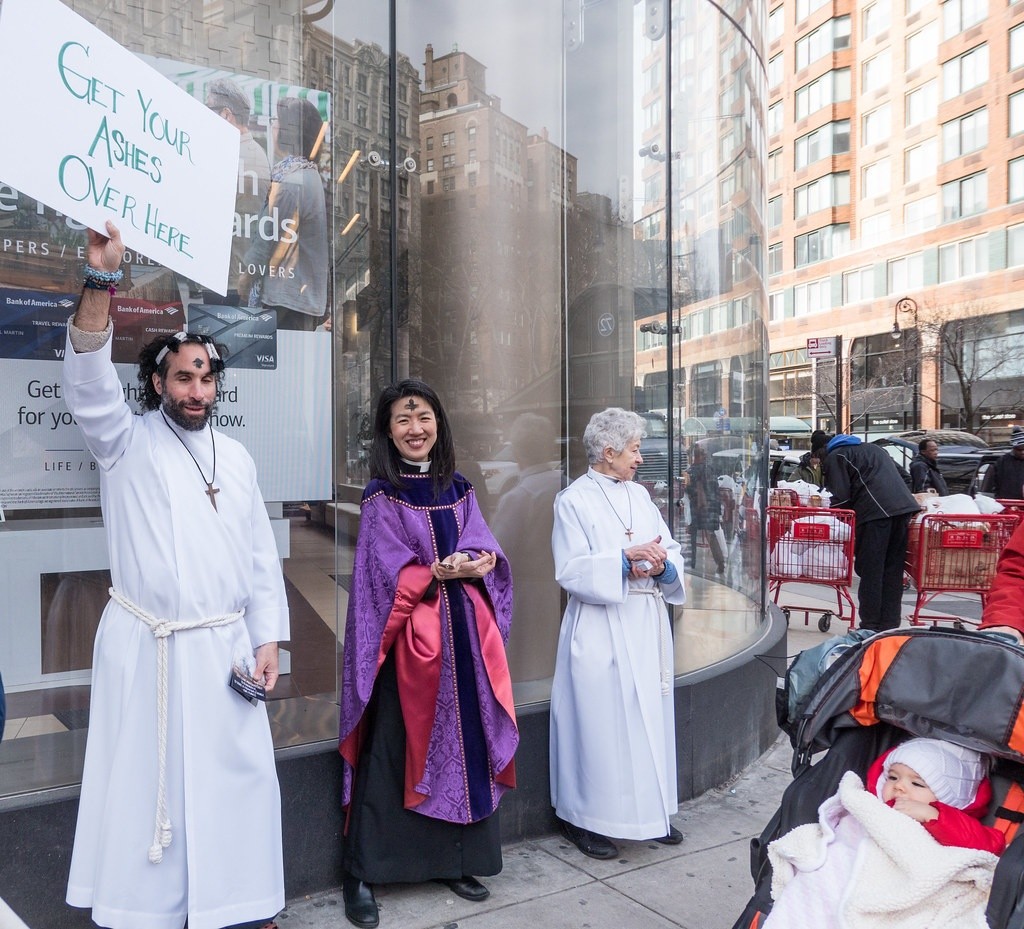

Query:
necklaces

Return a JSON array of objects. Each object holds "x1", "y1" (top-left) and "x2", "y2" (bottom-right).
[
  {"x1": 591, "y1": 474, "x2": 634, "y2": 543},
  {"x1": 160, "y1": 410, "x2": 219, "y2": 516},
  {"x1": 681, "y1": 445, "x2": 724, "y2": 572}
]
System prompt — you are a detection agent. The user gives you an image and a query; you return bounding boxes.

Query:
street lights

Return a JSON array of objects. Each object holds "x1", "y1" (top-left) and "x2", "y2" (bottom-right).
[{"x1": 882, "y1": 298, "x2": 918, "y2": 427}]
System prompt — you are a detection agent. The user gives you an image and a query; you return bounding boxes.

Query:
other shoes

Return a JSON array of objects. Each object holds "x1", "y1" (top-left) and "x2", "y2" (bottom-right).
[
  {"x1": 652, "y1": 824, "x2": 685, "y2": 845},
  {"x1": 559, "y1": 820, "x2": 619, "y2": 860},
  {"x1": 716, "y1": 563, "x2": 725, "y2": 574}
]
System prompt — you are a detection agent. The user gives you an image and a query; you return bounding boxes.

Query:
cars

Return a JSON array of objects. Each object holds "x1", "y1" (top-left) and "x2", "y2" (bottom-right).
[
  {"x1": 869, "y1": 428, "x2": 987, "y2": 476},
  {"x1": 633, "y1": 410, "x2": 691, "y2": 480},
  {"x1": 768, "y1": 449, "x2": 815, "y2": 486}
]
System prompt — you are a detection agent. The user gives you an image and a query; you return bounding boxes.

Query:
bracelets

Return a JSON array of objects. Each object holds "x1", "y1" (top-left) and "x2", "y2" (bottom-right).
[
  {"x1": 651, "y1": 567, "x2": 658, "y2": 576},
  {"x1": 84, "y1": 265, "x2": 123, "y2": 296}
]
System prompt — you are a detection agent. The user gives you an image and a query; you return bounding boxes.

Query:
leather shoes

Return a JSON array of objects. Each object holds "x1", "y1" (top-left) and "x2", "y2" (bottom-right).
[
  {"x1": 341, "y1": 873, "x2": 381, "y2": 928},
  {"x1": 438, "y1": 870, "x2": 490, "y2": 901}
]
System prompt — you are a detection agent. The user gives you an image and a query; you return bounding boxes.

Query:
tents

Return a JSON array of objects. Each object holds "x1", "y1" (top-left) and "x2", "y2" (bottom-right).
[{"x1": 166, "y1": 61, "x2": 330, "y2": 162}]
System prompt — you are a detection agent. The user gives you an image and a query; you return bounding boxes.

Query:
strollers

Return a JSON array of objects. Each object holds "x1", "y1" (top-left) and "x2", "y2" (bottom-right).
[{"x1": 733, "y1": 628, "x2": 1024, "y2": 929}]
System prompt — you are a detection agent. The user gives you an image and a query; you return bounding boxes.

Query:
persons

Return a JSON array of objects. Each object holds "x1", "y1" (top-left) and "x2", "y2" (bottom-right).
[
  {"x1": 811, "y1": 430, "x2": 922, "y2": 631},
  {"x1": 994, "y1": 425, "x2": 1024, "y2": 499},
  {"x1": 790, "y1": 451, "x2": 823, "y2": 488},
  {"x1": 489, "y1": 413, "x2": 576, "y2": 555},
  {"x1": 755, "y1": 738, "x2": 1005, "y2": 929},
  {"x1": 237, "y1": 97, "x2": 327, "y2": 331},
  {"x1": 976, "y1": 518, "x2": 1024, "y2": 645},
  {"x1": 62, "y1": 222, "x2": 287, "y2": 929},
  {"x1": 447, "y1": 420, "x2": 489, "y2": 520},
  {"x1": 909, "y1": 439, "x2": 949, "y2": 496},
  {"x1": 552, "y1": 407, "x2": 686, "y2": 860},
  {"x1": 337, "y1": 382, "x2": 520, "y2": 928},
  {"x1": 205, "y1": 79, "x2": 272, "y2": 304}
]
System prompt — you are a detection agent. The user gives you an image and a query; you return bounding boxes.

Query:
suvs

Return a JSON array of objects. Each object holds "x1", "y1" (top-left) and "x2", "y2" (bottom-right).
[{"x1": 931, "y1": 444, "x2": 1012, "y2": 499}]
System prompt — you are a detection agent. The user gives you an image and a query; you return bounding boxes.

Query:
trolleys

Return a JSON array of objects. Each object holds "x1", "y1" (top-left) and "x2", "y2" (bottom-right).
[
  {"x1": 718, "y1": 488, "x2": 856, "y2": 634},
  {"x1": 906, "y1": 492, "x2": 1024, "y2": 628}
]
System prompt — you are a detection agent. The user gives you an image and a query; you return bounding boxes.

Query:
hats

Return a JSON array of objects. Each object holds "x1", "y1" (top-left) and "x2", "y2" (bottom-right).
[
  {"x1": 1011, "y1": 424, "x2": 1024, "y2": 449},
  {"x1": 879, "y1": 736, "x2": 994, "y2": 811},
  {"x1": 687, "y1": 444, "x2": 707, "y2": 457},
  {"x1": 809, "y1": 429, "x2": 833, "y2": 453}
]
